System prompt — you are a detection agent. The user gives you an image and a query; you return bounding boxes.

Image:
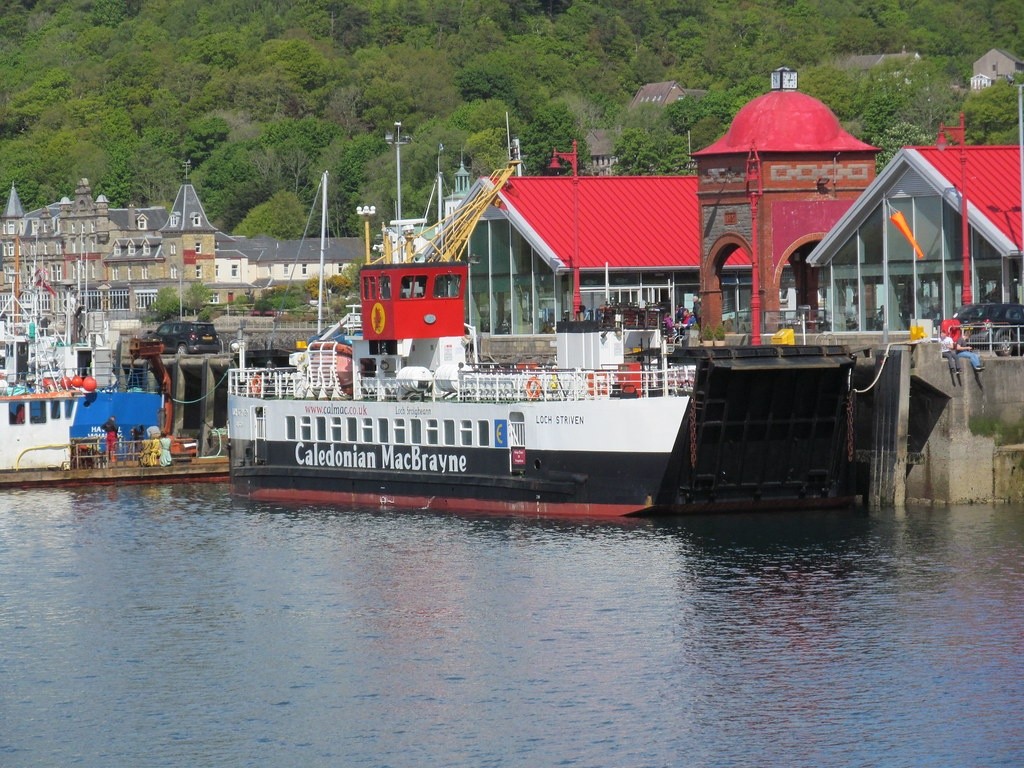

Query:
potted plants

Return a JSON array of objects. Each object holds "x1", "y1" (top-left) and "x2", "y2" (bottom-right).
[{"x1": 684, "y1": 321, "x2": 726, "y2": 347}]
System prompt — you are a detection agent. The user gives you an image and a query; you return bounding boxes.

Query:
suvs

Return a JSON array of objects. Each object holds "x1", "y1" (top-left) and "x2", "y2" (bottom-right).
[
  {"x1": 141, "y1": 320, "x2": 221, "y2": 355},
  {"x1": 953, "y1": 302, "x2": 1024, "y2": 357}
]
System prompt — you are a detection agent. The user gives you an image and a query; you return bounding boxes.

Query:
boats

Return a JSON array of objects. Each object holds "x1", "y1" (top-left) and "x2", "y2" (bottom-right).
[
  {"x1": 0, "y1": 224, "x2": 229, "y2": 488},
  {"x1": 225, "y1": 138, "x2": 954, "y2": 517}
]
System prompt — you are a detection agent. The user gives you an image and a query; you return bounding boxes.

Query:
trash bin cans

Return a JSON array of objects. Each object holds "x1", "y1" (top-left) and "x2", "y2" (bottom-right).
[{"x1": 772, "y1": 329, "x2": 796, "y2": 345}]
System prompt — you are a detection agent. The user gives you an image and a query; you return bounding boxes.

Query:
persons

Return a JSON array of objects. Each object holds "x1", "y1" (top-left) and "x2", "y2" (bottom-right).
[
  {"x1": 483, "y1": 319, "x2": 512, "y2": 335},
  {"x1": 158, "y1": 430, "x2": 172, "y2": 466},
  {"x1": 900, "y1": 297, "x2": 912, "y2": 329},
  {"x1": 129, "y1": 424, "x2": 144, "y2": 452},
  {"x1": 102, "y1": 416, "x2": 118, "y2": 459},
  {"x1": 664, "y1": 295, "x2": 701, "y2": 337},
  {"x1": 16, "y1": 405, "x2": 24, "y2": 424},
  {"x1": 927, "y1": 306, "x2": 937, "y2": 325},
  {"x1": 956, "y1": 331, "x2": 985, "y2": 371},
  {"x1": 938, "y1": 330, "x2": 963, "y2": 373},
  {"x1": 876, "y1": 305, "x2": 884, "y2": 331}
]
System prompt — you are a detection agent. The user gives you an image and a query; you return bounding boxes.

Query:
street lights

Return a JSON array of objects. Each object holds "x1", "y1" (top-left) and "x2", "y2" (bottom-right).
[
  {"x1": 385, "y1": 122, "x2": 412, "y2": 235},
  {"x1": 743, "y1": 137, "x2": 764, "y2": 346},
  {"x1": 356, "y1": 205, "x2": 376, "y2": 264},
  {"x1": 550, "y1": 139, "x2": 582, "y2": 321},
  {"x1": 934, "y1": 112, "x2": 972, "y2": 305}
]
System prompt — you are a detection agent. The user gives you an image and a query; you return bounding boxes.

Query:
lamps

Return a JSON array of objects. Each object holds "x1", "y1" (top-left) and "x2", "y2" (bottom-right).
[{"x1": 817, "y1": 179, "x2": 830, "y2": 192}]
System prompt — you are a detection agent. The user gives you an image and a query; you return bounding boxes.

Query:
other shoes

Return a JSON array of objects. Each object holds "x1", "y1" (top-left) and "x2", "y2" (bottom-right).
[
  {"x1": 950, "y1": 368, "x2": 963, "y2": 374},
  {"x1": 975, "y1": 366, "x2": 986, "y2": 370}
]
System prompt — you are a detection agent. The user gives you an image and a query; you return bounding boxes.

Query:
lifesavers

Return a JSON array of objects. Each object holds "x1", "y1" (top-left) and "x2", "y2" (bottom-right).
[
  {"x1": 526, "y1": 377, "x2": 542, "y2": 397},
  {"x1": 250, "y1": 375, "x2": 261, "y2": 394}
]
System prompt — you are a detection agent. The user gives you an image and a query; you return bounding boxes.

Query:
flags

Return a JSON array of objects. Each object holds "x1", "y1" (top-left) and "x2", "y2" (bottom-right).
[{"x1": 35, "y1": 274, "x2": 56, "y2": 297}]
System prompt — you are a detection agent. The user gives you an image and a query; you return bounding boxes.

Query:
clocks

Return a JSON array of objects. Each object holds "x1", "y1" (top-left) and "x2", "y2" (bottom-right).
[{"x1": 771, "y1": 67, "x2": 798, "y2": 90}]
